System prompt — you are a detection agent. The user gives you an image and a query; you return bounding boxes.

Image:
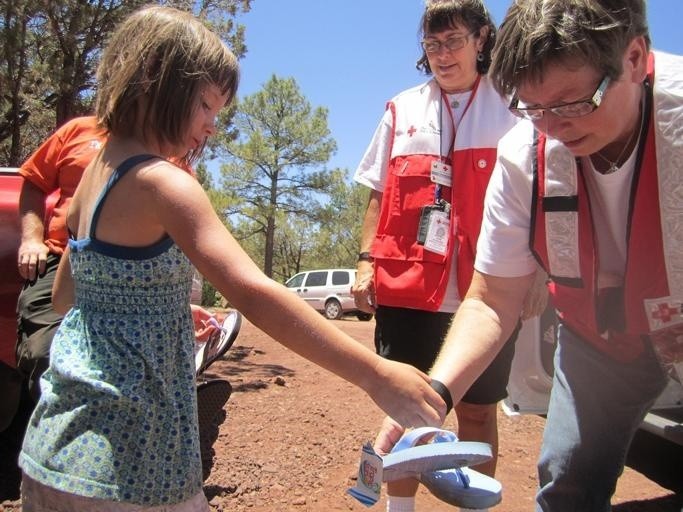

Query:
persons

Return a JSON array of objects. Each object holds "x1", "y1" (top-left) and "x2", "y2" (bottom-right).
[
  {"x1": 372, "y1": 1, "x2": 682, "y2": 511},
  {"x1": 350, "y1": 0, "x2": 551, "y2": 511},
  {"x1": 17, "y1": 0, "x2": 448, "y2": 512},
  {"x1": 17, "y1": 116, "x2": 198, "y2": 445}
]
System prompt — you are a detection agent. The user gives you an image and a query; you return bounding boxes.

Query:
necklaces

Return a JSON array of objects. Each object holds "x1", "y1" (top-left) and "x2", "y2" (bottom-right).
[
  {"x1": 444, "y1": 84, "x2": 471, "y2": 108},
  {"x1": 594, "y1": 128, "x2": 634, "y2": 174}
]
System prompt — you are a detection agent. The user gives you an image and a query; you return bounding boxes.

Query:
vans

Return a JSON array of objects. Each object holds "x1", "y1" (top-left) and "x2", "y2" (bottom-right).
[{"x1": 284, "y1": 269, "x2": 373, "y2": 320}]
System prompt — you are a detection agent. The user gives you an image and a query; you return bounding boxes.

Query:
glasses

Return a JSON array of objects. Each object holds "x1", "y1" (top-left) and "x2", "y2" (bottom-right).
[
  {"x1": 421, "y1": 30, "x2": 475, "y2": 52},
  {"x1": 508, "y1": 76, "x2": 610, "y2": 120}
]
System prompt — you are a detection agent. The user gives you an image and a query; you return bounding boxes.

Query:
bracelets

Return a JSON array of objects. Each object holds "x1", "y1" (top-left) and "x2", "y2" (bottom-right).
[
  {"x1": 428, "y1": 378, "x2": 453, "y2": 415},
  {"x1": 359, "y1": 252, "x2": 374, "y2": 263}
]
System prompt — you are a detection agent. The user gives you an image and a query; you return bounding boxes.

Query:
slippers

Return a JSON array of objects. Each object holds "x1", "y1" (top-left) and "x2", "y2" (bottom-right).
[
  {"x1": 196, "y1": 309, "x2": 242, "y2": 428},
  {"x1": 383, "y1": 427, "x2": 502, "y2": 510}
]
